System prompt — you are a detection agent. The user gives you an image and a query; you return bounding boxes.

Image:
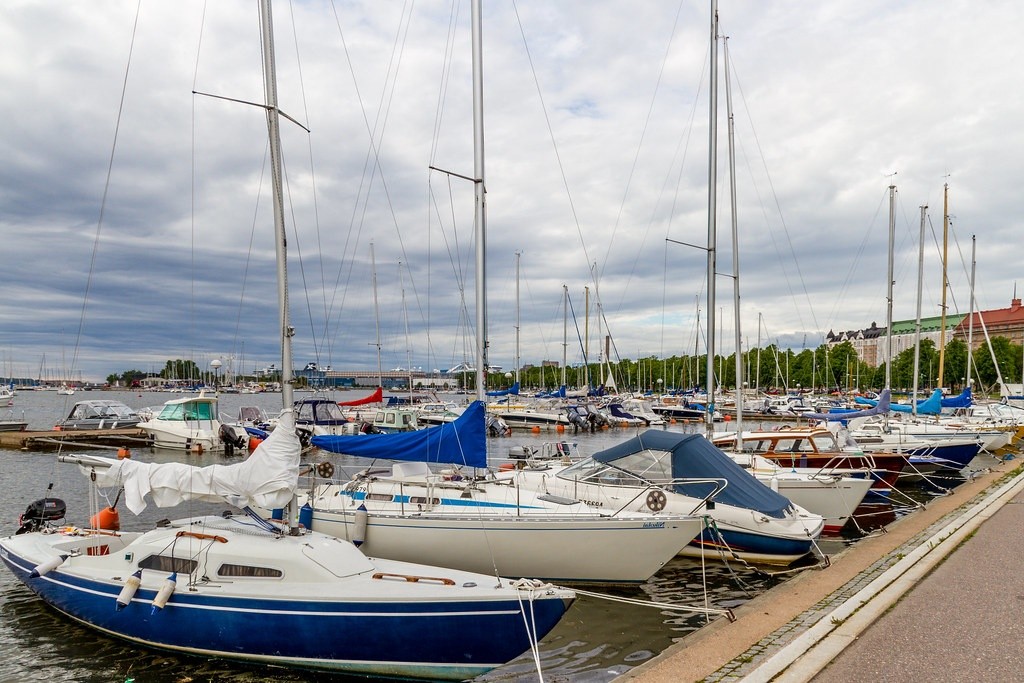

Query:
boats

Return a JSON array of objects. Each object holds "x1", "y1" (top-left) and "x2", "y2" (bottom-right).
[
  {"x1": 137, "y1": 405, "x2": 208, "y2": 423},
  {"x1": 137, "y1": 397, "x2": 249, "y2": 454},
  {"x1": 0, "y1": 387, "x2": 14, "y2": 406},
  {"x1": 53, "y1": 399, "x2": 142, "y2": 429},
  {"x1": 57, "y1": 382, "x2": 75, "y2": 396},
  {"x1": 0, "y1": 408, "x2": 28, "y2": 431}
]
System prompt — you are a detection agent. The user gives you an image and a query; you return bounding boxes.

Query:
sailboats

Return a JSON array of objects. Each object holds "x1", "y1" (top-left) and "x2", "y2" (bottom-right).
[
  {"x1": 244, "y1": 0, "x2": 1023, "y2": 588},
  {"x1": 1, "y1": 345, "x2": 260, "y2": 394},
  {"x1": 0, "y1": 0, "x2": 578, "y2": 680}
]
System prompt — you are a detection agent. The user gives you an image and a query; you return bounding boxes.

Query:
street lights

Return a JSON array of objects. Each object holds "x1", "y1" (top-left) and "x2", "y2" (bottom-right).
[{"x1": 209, "y1": 359, "x2": 223, "y2": 421}]
